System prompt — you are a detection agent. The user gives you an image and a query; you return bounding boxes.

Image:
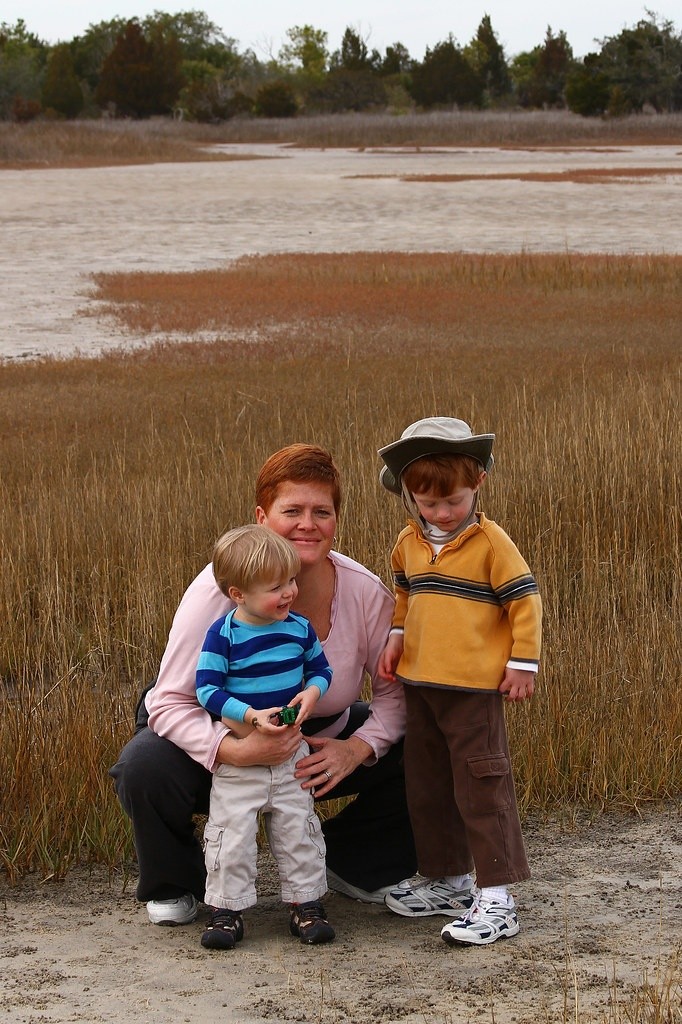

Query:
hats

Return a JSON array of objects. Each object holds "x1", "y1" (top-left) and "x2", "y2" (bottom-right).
[{"x1": 377, "y1": 417, "x2": 496, "y2": 498}]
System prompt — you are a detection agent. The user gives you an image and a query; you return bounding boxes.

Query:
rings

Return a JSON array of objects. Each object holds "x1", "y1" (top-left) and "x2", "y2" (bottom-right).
[{"x1": 325, "y1": 771, "x2": 332, "y2": 779}]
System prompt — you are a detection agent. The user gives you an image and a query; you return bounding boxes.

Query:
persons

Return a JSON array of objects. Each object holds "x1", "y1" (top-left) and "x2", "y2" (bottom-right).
[
  {"x1": 108, "y1": 444, "x2": 415, "y2": 926},
  {"x1": 379, "y1": 417, "x2": 541, "y2": 945},
  {"x1": 195, "y1": 525, "x2": 334, "y2": 952}
]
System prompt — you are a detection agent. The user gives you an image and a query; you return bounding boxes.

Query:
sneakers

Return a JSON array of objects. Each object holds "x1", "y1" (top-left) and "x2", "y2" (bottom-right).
[
  {"x1": 384, "y1": 875, "x2": 478, "y2": 917},
  {"x1": 324, "y1": 866, "x2": 414, "y2": 903},
  {"x1": 202, "y1": 908, "x2": 244, "y2": 948},
  {"x1": 290, "y1": 901, "x2": 334, "y2": 943},
  {"x1": 440, "y1": 887, "x2": 520, "y2": 946},
  {"x1": 146, "y1": 891, "x2": 198, "y2": 926}
]
